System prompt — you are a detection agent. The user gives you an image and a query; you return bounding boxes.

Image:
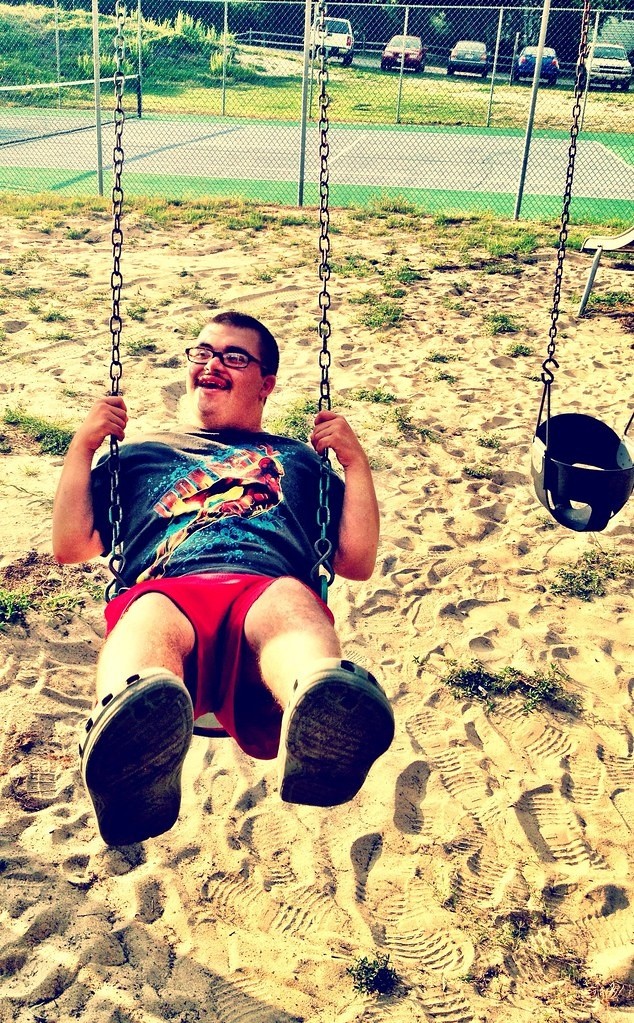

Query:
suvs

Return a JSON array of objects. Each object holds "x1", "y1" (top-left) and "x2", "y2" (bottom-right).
[
  {"x1": 310, "y1": 16, "x2": 360, "y2": 67},
  {"x1": 577, "y1": 43, "x2": 634, "y2": 93}
]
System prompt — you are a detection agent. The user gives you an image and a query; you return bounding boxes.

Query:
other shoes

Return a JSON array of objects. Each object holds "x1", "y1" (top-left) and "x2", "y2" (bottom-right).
[
  {"x1": 276, "y1": 658, "x2": 396, "y2": 808},
  {"x1": 77, "y1": 667, "x2": 195, "y2": 846}
]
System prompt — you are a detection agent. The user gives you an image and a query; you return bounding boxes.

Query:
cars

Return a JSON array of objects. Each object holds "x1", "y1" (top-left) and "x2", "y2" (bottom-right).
[
  {"x1": 512, "y1": 44, "x2": 560, "y2": 86},
  {"x1": 381, "y1": 34, "x2": 429, "y2": 73},
  {"x1": 447, "y1": 40, "x2": 495, "y2": 76}
]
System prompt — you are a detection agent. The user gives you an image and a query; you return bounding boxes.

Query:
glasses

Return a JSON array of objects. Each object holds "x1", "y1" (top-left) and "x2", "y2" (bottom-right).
[{"x1": 185, "y1": 347, "x2": 263, "y2": 368}]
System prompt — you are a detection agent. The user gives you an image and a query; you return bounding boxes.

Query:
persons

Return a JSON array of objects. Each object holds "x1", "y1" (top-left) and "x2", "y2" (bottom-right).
[{"x1": 51, "y1": 311, "x2": 396, "y2": 847}]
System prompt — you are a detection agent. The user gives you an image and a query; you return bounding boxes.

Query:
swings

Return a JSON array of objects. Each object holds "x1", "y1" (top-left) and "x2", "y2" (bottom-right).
[
  {"x1": 107, "y1": 0, "x2": 337, "y2": 738},
  {"x1": 526, "y1": 1, "x2": 634, "y2": 534}
]
